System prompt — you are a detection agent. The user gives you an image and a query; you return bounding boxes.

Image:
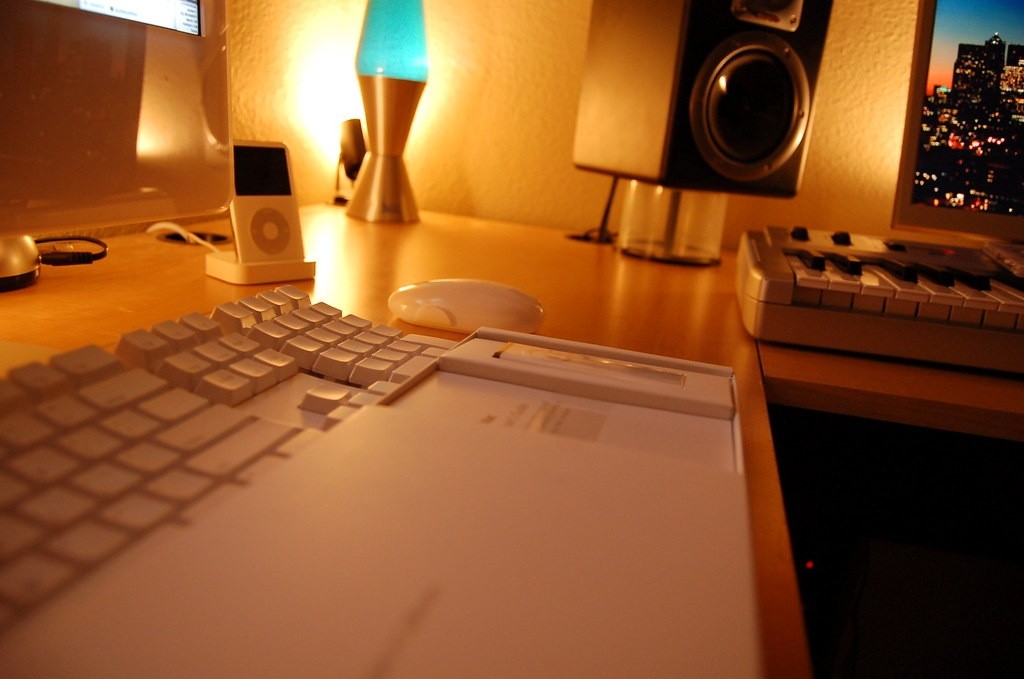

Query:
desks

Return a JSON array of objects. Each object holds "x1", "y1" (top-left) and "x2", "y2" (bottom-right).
[{"x1": 0, "y1": 198, "x2": 1024, "y2": 679}]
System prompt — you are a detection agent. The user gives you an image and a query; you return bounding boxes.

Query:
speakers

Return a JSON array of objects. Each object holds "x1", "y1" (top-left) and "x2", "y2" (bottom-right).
[{"x1": 572, "y1": 0, "x2": 834, "y2": 199}]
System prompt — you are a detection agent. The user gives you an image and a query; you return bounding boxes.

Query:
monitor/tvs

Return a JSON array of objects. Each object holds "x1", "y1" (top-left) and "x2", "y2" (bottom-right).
[
  {"x1": 891, "y1": 0, "x2": 1024, "y2": 249},
  {"x1": 0, "y1": 0, "x2": 229, "y2": 236}
]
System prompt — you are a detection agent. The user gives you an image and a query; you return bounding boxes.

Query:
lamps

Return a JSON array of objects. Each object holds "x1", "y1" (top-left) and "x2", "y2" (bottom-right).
[{"x1": 331, "y1": 119, "x2": 368, "y2": 208}]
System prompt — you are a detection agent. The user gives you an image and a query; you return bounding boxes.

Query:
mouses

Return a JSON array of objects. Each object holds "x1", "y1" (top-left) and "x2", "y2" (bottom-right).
[{"x1": 389, "y1": 278, "x2": 545, "y2": 335}]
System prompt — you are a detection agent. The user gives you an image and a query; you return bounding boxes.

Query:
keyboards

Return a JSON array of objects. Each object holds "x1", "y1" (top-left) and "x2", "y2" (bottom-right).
[{"x1": 0, "y1": 285, "x2": 461, "y2": 640}]
